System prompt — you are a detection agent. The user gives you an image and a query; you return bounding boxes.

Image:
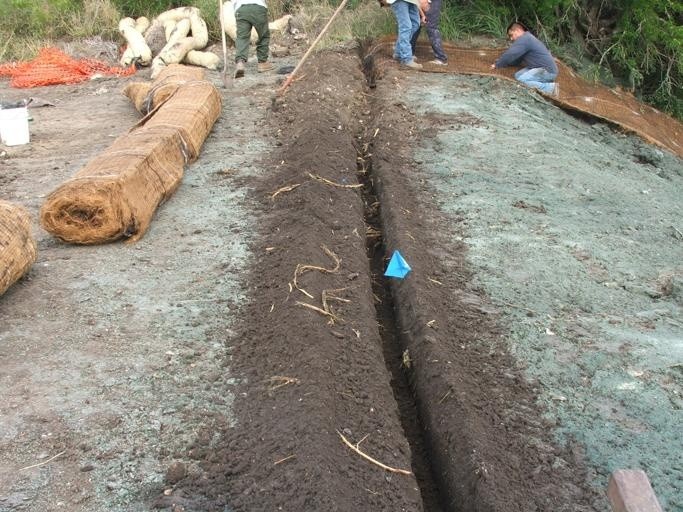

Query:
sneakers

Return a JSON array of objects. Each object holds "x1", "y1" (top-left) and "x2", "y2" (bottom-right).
[
  {"x1": 393, "y1": 53, "x2": 417, "y2": 61},
  {"x1": 398, "y1": 61, "x2": 423, "y2": 71},
  {"x1": 552, "y1": 83, "x2": 559, "y2": 100},
  {"x1": 257, "y1": 61, "x2": 277, "y2": 73},
  {"x1": 235, "y1": 62, "x2": 244, "y2": 78},
  {"x1": 428, "y1": 60, "x2": 449, "y2": 66}
]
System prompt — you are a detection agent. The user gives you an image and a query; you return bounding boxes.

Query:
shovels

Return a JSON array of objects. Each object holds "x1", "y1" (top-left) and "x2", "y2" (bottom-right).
[{"x1": 220, "y1": 0, "x2": 234, "y2": 89}]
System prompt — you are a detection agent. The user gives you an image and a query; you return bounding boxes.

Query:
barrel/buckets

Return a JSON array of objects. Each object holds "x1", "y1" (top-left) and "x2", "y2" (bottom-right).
[{"x1": 0, "y1": 101, "x2": 30, "y2": 146}]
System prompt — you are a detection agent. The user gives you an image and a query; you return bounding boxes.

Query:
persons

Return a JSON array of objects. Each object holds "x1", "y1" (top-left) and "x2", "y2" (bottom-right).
[
  {"x1": 231, "y1": 0, "x2": 276, "y2": 78},
  {"x1": 384, "y1": 0, "x2": 432, "y2": 68},
  {"x1": 491, "y1": 22, "x2": 560, "y2": 98},
  {"x1": 410, "y1": 0, "x2": 448, "y2": 66}
]
